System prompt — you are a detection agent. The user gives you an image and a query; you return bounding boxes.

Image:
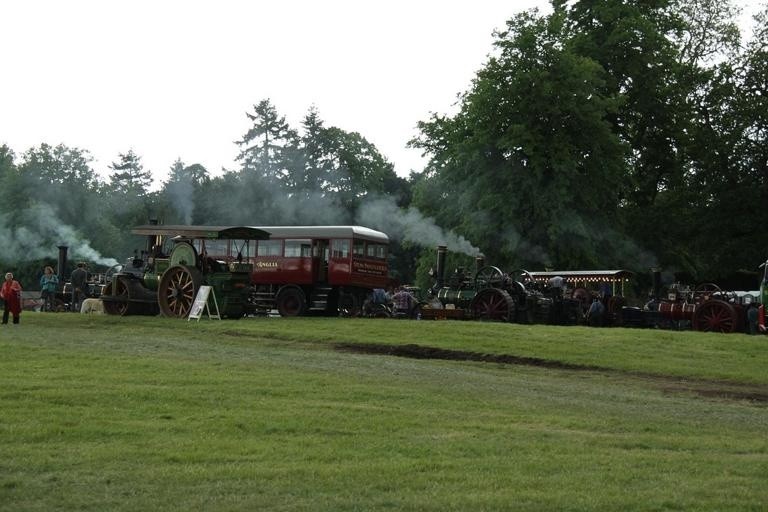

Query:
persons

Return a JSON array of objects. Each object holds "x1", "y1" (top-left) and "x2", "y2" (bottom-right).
[
  {"x1": 70, "y1": 261, "x2": 88, "y2": 313},
  {"x1": 547, "y1": 275, "x2": 567, "y2": 301},
  {"x1": 0, "y1": 272, "x2": 23, "y2": 325},
  {"x1": 385, "y1": 285, "x2": 422, "y2": 318},
  {"x1": 585, "y1": 295, "x2": 605, "y2": 327},
  {"x1": 39, "y1": 266, "x2": 59, "y2": 312}
]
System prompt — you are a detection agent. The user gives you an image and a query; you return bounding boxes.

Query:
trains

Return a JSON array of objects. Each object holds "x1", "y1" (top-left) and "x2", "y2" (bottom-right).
[
  {"x1": 369, "y1": 245, "x2": 768, "y2": 333},
  {"x1": 98, "y1": 219, "x2": 273, "y2": 322},
  {"x1": 43, "y1": 245, "x2": 113, "y2": 312}
]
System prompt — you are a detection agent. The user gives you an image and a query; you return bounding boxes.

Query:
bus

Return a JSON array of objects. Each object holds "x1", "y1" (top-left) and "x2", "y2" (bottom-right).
[{"x1": 192, "y1": 225, "x2": 390, "y2": 318}]
